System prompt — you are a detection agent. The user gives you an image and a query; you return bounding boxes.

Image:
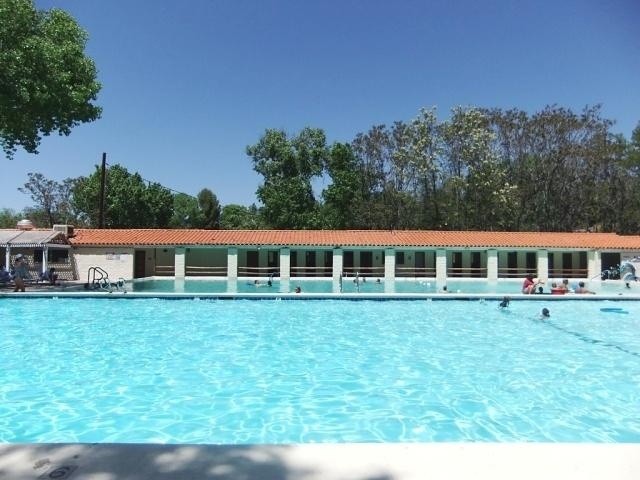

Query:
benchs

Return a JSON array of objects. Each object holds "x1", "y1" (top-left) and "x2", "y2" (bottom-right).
[{"x1": 0, "y1": 278, "x2": 67, "y2": 289}]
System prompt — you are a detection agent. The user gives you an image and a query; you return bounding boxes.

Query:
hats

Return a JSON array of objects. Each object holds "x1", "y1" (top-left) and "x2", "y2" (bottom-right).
[{"x1": 15, "y1": 254, "x2": 22, "y2": 259}]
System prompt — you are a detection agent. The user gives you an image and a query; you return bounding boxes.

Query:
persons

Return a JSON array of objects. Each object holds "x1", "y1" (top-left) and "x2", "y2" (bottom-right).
[
  {"x1": 295, "y1": 286, "x2": 304, "y2": 293},
  {"x1": 575, "y1": 281, "x2": 596, "y2": 294},
  {"x1": 375, "y1": 279, "x2": 381, "y2": 284},
  {"x1": 254, "y1": 280, "x2": 266, "y2": 287},
  {"x1": 551, "y1": 283, "x2": 559, "y2": 289},
  {"x1": 561, "y1": 280, "x2": 575, "y2": 294},
  {"x1": 267, "y1": 281, "x2": 272, "y2": 287},
  {"x1": 353, "y1": 279, "x2": 358, "y2": 286},
  {"x1": 41, "y1": 267, "x2": 55, "y2": 286},
  {"x1": 0, "y1": 258, "x2": 28, "y2": 292},
  {"x1": 521, "y1": 273, "x2": 539, "y2": 295}
]
